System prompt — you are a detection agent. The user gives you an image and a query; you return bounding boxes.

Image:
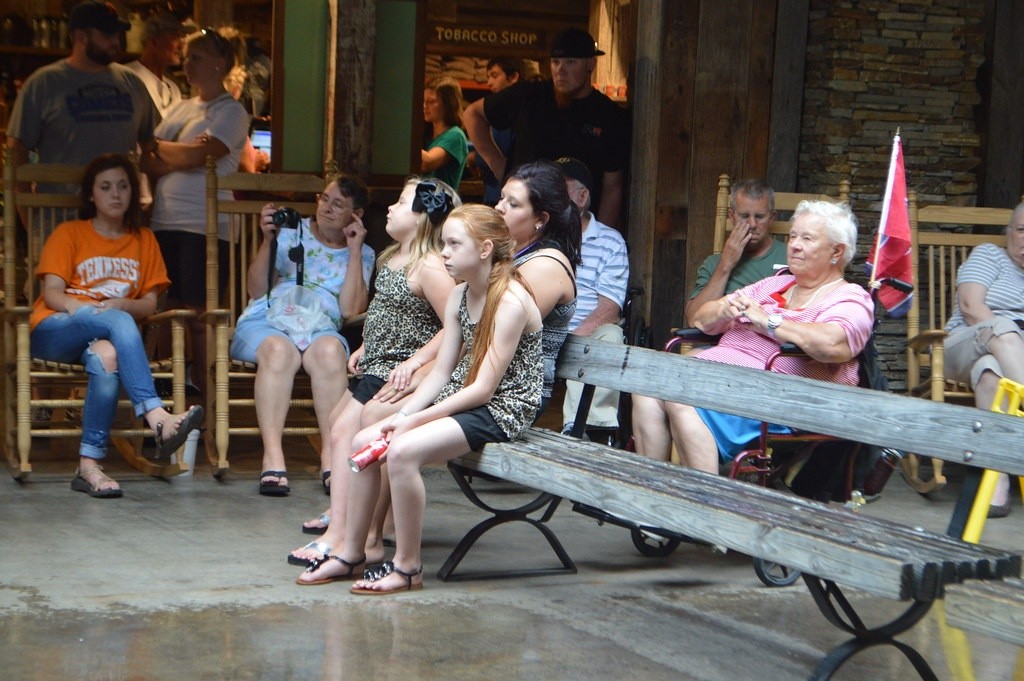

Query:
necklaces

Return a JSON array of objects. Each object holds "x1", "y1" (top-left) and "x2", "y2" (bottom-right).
[{"x1": 787, "y1": 276, "x2": 844, "y2": 308}]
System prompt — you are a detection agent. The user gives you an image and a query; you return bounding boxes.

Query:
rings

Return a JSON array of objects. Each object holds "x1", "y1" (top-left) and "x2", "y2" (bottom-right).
[{"x1": 394, "y1": 387, "x2": 398, "y2": 394}]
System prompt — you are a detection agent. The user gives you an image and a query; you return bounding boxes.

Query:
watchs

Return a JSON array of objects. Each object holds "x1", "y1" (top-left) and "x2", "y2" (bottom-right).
[{"x1": 767, "y1": 314, "x2": 784, "y2": 340}]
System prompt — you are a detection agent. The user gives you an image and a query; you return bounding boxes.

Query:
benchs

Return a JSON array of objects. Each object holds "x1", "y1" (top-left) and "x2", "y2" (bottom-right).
[
  {"x1": 438, "y1": 332, "x2": 1024, "y2": 681},
  {"x1": 943, "y1": 575, "x2": 1024, "y2": 648}
]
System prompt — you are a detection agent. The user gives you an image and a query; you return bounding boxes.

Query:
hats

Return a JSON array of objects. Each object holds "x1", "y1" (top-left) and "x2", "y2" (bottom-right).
[
  {"x1": 140, "y1": 12, "x2": 197, "y2": 41},
  {"x1": 551, "y1": 157, "x2": 595, "y2": 196},
  {"x1": 551, "y1": 29, "x2": 605, "y2": 57},
  {"x1": 68, "y1": 0, "x2": 131, "y2": 32}
]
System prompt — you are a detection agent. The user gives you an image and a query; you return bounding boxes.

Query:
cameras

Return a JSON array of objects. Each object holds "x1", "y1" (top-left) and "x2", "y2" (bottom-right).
[{"x1": 268, "y1": 208, "x2": 301, "y2": 229}]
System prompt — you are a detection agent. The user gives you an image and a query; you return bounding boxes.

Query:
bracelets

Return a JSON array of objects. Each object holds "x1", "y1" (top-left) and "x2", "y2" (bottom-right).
[{"x1": 399, "y1": 410, "x2": 408, "y2": 417}]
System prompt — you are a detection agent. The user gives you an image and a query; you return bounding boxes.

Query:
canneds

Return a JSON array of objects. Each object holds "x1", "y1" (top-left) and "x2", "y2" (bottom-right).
[{"x1": 348, "y1": 437, "x2": 389, "y2": 474}]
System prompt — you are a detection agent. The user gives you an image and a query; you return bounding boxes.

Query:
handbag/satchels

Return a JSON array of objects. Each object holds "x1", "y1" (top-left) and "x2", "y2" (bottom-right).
[{"x1": 267, "y1": 284, "x2": 336, "y2": 352}]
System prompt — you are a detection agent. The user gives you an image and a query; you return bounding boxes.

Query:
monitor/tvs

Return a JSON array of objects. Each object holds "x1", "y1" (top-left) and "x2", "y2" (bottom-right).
[{"x1": 248, "y1": 116, "x2": 271, "y2": 158}]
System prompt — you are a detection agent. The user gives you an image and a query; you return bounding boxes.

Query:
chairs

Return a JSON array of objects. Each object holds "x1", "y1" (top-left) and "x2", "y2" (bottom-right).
[
  {"x1": 672, "y1": 175, "x2": 852, "y2": 467},
  {"x1": 0, "y1": 148, "x2": 196, "y2": 480},
  {"x1": 899, "y1": 188, "x2": 1024, "y2": 493},
  {"x1": 199, "y1": 155, "x2": 341, "y2": 477}
]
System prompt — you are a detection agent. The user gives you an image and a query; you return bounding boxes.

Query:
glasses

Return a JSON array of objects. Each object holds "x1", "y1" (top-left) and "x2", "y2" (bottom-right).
[
  {"x1": 201, "y1": 24, "x2": 232, "y2": 56},
  {"x1": 1011, "y1": 224, "x2": 1024, "y2": 232},
  {"x1": 161, "y1": 80, "x2": 173, "y2": 108},
  {"x1": 316, "y1": 192, "x2": 358, "y2": 214}
]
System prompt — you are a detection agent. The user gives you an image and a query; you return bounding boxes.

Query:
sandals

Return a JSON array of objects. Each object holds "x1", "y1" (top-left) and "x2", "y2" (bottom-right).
[
  {"x1": 297, "y1": 553, "x2": 367, "y2": 585},
  {"x1": 352, "y1": 560, "x2": 425, "y2": 596},
  {"x1": 154, "y1": 404, "x2": 205, "y2": 461},
  {"x1": 71, "y1": 463, "x2": 122, "y2": 499}
]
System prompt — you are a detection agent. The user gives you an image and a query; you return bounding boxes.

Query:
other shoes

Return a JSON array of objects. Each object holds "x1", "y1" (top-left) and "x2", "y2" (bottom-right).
[
  {"x1": 561, "y1": 423, "x2": 590, "y2": 442},
  {"x1": 35, "y1": 408, "x2": 83, "y2": 424},
  {"x1": 988, "y1": 487, "x2": 1011, "y2": 519}
]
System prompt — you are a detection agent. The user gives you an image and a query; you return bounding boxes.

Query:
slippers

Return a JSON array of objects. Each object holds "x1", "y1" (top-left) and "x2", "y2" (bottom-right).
[
  {"x1": 287, "y1": 540, "x2": 334, "y2": 566},
  {"x1": 321, "y1": 470, "x2": 331, "y2": 493},
  {"x1": 382, "y1": 538, "x2": 395, "y2": 547},
  {"x1": 302, "y1": 512, "x2": 331, "y2": 534},
  {"x1": 259, "y1": 471, "x2": 291, "y2": 495}
]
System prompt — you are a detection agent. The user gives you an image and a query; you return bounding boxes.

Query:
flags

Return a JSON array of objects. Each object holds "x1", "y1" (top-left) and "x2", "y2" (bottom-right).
[{"x1": 865, "y1": 135, "x2": 915, "y2": 317}]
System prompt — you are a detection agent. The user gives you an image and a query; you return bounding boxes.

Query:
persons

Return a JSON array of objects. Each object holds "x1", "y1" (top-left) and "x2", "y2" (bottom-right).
[
  {"x1": 630, "y1": 200, "x2": 874, "y2": 476},
  {"x1": 929, "y1": 199, "x2": 1024, "y2": 517},
  {"x1": 28, "y1": 153, "x2": 204, "y2": 498},
  {"x1": 287, "y1": 27, "x2": 629, "y2": 595},
  {"x1": 5, "y1": 0, "x2": 260, "y2": 442},
  {"x1": 229, "y1": 173, "x2": 375, "y2": 493},
  {"x1": 683, "y1": 179, "x2": 789, "y2": 356}
]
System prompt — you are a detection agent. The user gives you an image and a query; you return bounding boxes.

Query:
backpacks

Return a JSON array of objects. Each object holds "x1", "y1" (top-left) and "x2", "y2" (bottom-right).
[{"x1": 833, "y1": 343, "x2": 905, "y2": 504}]
